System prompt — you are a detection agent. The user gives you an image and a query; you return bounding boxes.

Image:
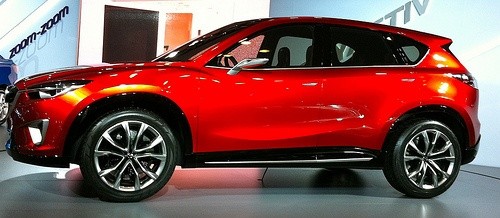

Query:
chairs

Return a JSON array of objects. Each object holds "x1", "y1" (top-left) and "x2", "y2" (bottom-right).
[
  {"x1": 302, "y1": 46, "x2": 314, "y2": 68},
  {"x1": 275, "y1": 46, "x2": 291, "y2": 67}
]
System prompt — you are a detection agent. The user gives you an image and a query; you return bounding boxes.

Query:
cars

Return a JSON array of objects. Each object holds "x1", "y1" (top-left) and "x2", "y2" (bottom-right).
[
  {"x1": 5, "y1": 15, "x2": 481, "y2": 202},
  {"x1": 0, "y1": 55, "x2": 18, "y2": 125}
]
